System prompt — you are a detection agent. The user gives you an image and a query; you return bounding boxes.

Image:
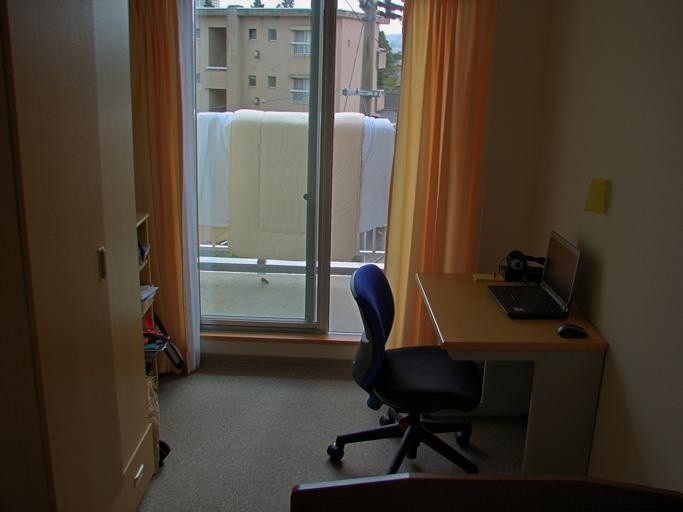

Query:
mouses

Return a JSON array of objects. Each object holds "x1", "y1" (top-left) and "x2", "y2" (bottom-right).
[{"x1": 558, "y1": 323, "x2": 588, "y2": 338}]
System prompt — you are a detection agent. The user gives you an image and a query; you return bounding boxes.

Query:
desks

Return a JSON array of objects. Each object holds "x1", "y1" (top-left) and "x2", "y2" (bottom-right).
[{"x1": 416, "y1": 272, "x2": 609, "y2": 479}]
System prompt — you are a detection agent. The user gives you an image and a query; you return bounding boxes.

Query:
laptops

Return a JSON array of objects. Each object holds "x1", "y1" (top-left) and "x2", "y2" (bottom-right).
[{"x1": 487, "y1": 230, "x2": 581, "y2": 319}]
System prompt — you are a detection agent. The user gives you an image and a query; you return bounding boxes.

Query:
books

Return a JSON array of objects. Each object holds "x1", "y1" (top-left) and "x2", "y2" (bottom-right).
[{"x1": 472, "y1": 273, "x2": 505, "y2": 282}]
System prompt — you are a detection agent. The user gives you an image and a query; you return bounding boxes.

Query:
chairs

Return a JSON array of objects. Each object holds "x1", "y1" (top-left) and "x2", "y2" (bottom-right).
[{"x1": 327, "y1": 265, "x2": 484, "y2": 474}]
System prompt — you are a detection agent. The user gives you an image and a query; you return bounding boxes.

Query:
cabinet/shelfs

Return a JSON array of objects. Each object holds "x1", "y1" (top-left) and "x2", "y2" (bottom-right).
[
  {"x1": 8, "y1": 0, "x2": 157, "y2": 512},
  {"x1": 137, "y1": 212, "x2": 155, "y2": 385}
]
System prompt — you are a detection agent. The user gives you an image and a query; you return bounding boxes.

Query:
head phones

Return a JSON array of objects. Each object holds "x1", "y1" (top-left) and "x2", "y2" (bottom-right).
[{"x1": 506, "y1": 250, "x2": 545, "y2": 270}]
